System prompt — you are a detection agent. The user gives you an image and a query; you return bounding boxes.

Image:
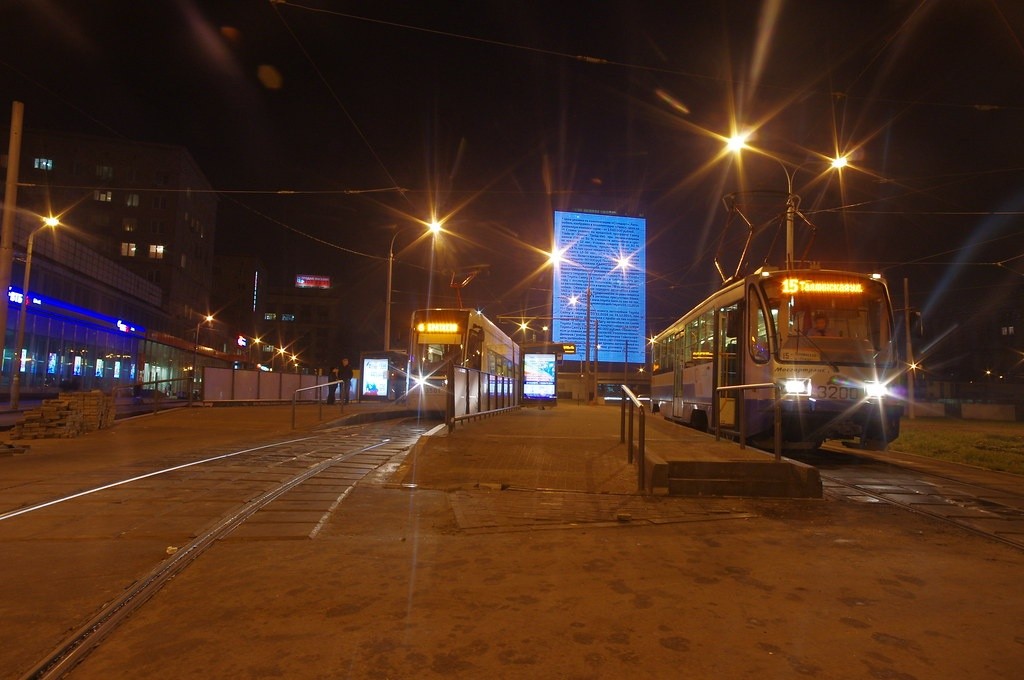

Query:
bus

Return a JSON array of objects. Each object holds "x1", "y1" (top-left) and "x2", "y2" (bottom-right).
[
  {"x1": 404, "y1": 308, "x2": 523, "y2": 419},
  {"x1": 645, "y1": 266, "x2": 926, "y2": 456}
]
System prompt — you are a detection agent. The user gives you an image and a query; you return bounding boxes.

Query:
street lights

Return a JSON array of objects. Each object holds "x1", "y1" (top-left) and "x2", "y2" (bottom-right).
[
  {"x1": 550, "y1": 253, "x2": 633, "y2": 405},
  {"x1": 385, "y1": 220, "x2": 443, "y2": 352},
  {"x1": 726, "y1": 130, "x2": 848, "y2": 461},
  {"x1": 11, "y1": 217, "x2": 60, "y2": 410}
]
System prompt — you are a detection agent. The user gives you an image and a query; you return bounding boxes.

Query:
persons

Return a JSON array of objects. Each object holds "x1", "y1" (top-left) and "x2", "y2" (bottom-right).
[
  {"x1": 806, "y1": 314, "x2": 840, "y2": 339},
  {"x1": 325, "y1": 364, "x2": 339, "y2": 406},
  {"x1": 335, "y1": 358, "x2": 355, "y2": 402}
]
